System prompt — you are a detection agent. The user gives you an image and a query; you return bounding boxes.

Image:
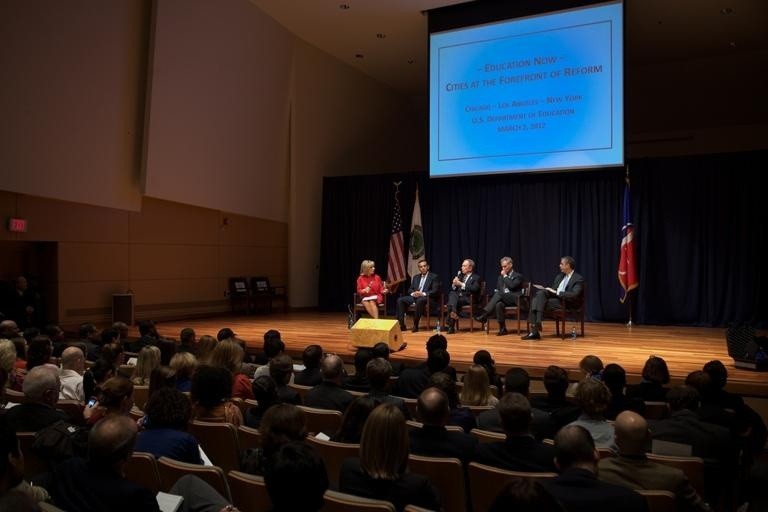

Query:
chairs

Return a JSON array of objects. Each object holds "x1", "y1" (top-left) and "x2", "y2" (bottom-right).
[
  {"x1": 644, "y1": 453, "x2": 705, "y2": 490},
  {"x1": 297, "y1": 406, "x2": 344, "y2": 437},
  {"x1": 446, "y1": 426, "x2": 465, "y2": 446},
  {"x1": 643, "y1": 402, "x2": 673, "y2": 421},
  {"x1": 528, "y1": 390, "x2": 549, "y2": 404},
  {"x1": 542, "y1": 438, "x2": 556, "y2": 461},
  {"x1": 133, "y1": 385, "x2": 151, "y2": 408},
  {"x1": 653, "y1": 440, "x2": 692, "y2": 457},
  {"x1": 286, "y1": 385, "x2": 316, "y2": 402},
  {"x1": 4, "y1": 389, "x2": 26, "y2": 405},
  {"x1": 120, "y1": 451, "x2": 158, "y2": 491},
  {"x1": 403, "y1": 399, "x2": 420, "y2": 418},
  {"x1": 49, "y1": 357, "x2": 62, "y2": 367},
  {"x1": 468, "y1": 462, "x2": 559, "y2": 510},
  {"x1": 353, "y1": 280, "x2": 585, "y2": 341},
  {"x1": 407, "y1": 455, "x2": 467, "y2": 509},
  {"x1": 120, "y1": 365, "x2": 137, "y2": 379},
  {"x1": 595, "y1": 447, "x2": 618, "y2": 460},
  {"x1": 129, "y1": 410, "x2": 145, "y2": 421},
  {"x1": 192, "y1": 421, "x2": 238, "y2": 470},
  {"x1": 230, "y1": 398, "x2": 257, "y2": 416},
  {"x1": 388, "y1": 376, "x2": 399, "y2": 390},
  {"x1": 304, "y1": 436, "x2": 361, "y2": 490},
  {"x1": 84, "y1": 361, "x2": 96, "y2": 370},
  {"x1": 237, "y1": 426, "x2": 267, "y2": 469},
  {"x1": 15, "y1": 432, "x2": 41, "y2": 458},
  {"x1": 460, "y1": 405, "x2": 497, "y2": 415},
  {"x1": 183, "y1": 391, "x2": 194, "y2": 410},
  {"x1": 228, "y1": 470, "x2": 265, "y2": 509},
  {"x1": 471, "y1": 429, "x2": 508, "y2": 456},
  {"x1": 405, "y1": 420, "x2": 424, "y2": 442},
  {"x1": 158, "y1": 457, "x2": 226, "y2": 496},
  {"x1": 637, "y1": 491, "x2": 677, "y2": 509},
  {"x1": 455, "y1": 381, "x2": 464, "y2": 393},
  {"x1": 56, "y1": 401, "x2": 85, "y2": 416},
  {"x1": 343, "y1": 374, "x2": 356, "y2": 385},
  {"x1": 347, "y1": 390, "x2": 370, "y2": 402},
  {"x1": 322, "y1": 491, "x2": 427, "y2": 512}
]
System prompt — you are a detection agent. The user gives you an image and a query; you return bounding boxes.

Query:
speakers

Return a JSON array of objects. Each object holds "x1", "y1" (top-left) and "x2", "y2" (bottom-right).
[
  {"x1": 111, "y1": 293, "x2": 135, "y2": 326},
  {"x1": 351, "y1": 317, "x2": 404, "y2": 352}
]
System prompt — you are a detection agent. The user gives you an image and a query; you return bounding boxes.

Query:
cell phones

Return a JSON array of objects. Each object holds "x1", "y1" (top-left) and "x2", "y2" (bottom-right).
[{"x1": 88, "y1": 396, "x2": 96, "y2": 408}]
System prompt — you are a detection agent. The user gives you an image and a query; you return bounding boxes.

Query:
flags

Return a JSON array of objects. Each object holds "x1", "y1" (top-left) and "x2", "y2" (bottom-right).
[
  {"x1": 406, "y1": 188, "x2": 426, "y2": 286},
  {"x1": 387, "y1": 188, "x2": 408, "y2": 295},
  {"x1": 615, "y1": 176, "x2": 640, "y2": 305}
]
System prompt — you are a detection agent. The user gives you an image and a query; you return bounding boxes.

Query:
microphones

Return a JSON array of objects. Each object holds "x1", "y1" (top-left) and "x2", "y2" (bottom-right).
[{"x1": 457, "y1": 271, "x2": 462, "y2": 278}]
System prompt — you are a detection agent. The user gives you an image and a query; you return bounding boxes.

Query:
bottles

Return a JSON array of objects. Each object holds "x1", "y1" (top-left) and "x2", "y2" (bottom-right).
[
  {"x1": 484, "y1": 322, "x2": 488, "y2": 334},
  {"x1": 571, "y1": 326, "x2": 576, "y2": 339},
  {"x1": 436, "y1": 320, "x2": 440, "y2": 333}
]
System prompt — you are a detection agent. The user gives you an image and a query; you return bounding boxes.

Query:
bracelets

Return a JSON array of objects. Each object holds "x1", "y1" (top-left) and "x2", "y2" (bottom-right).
[{"x1": 367, "y1": 285, "x2": 371, "y2": 289}]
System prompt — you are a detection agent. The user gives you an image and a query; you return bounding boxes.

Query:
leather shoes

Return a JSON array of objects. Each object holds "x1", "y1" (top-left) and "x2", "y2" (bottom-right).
[
  {"x1": 496, "y1": 327, "x2": 507, "y2": 335},
  {"x1": 520, "y1": 331, "x2": 540, "y2": 340},
  {"x1": 530, "y1": 322, "x2": 541, "y2": 331},
  {"x1": 473, "y1": 316, "x2": 488, "y2": 323}
]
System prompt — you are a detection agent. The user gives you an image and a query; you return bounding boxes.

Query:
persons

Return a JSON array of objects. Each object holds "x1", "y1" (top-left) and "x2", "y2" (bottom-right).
[
  {"x1": 8, "y1": 276, "x2": 34, "y2": 324},
  {"x1": 396, "y1": 260, "x2": 440, "y2": 332},
  {"x1": 520, "y1": 255, "x2": 585, "y2": 339},
  {"x1": 471, "y1": 256, "x2": 527, "y2": 336},
  {"x1": 446, "y1": 258, "x2": 481, "y2": 334},
  {"x1": 356, "y1": 260, "x2": 386, "y2": 319}
]
